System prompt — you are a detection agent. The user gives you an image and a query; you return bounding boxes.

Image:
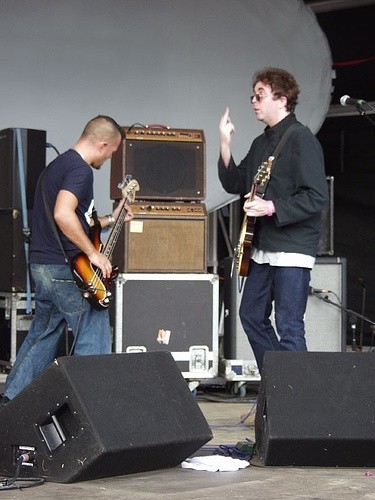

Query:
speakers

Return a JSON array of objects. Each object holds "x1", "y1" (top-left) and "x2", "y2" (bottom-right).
[
  {"x1": 0, "y1": 210, "x2": 40, "y2": 293},
  {"x1": 0, "y1": 351, "x2": 214, "y2": 483},
  {"x1": 224, "y1": 256, "x2": 350, "y2": 384},
  {"x1": 251, "y1": 350, "x2": 375, "y2": 469},
  {"x1": 0, "y1": 128, "x2": 45, "y2": 212},
  {"x1": 108, "y1": 126, "x2": 208, "y2": 202},
  {"x1": 111, "y1": 201, "x2": 209, "y2": 274}
]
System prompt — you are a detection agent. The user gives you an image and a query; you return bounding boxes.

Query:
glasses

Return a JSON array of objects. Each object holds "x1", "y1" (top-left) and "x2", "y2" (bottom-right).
[{"x1": 250, "y1": 94, "x2": 271, "y2": 104}]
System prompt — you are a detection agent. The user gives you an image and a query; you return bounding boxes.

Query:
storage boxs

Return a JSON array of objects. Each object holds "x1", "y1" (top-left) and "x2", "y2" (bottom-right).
[
  {"x1": 0, "y1": 292, "x2": 68, "y2": 368},
  {"x1": 108, "y1": 273, "x2": 219, "y2": 378}
]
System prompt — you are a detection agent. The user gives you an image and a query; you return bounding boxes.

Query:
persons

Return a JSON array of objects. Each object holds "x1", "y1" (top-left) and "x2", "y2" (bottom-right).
[
  {"x1": 217, "y1": 66, "x2": 329, "y2": 378},
  {"x1": 3, "y1": 114, "x2": 134, "y2": 401}
]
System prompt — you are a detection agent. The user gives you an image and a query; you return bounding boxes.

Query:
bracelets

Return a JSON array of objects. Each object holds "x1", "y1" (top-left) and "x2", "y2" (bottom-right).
[
  {"x1": 267, "y1": 200, "x2": 272, "y2": 217},
  {"x1": 105, "y1": 214, "x2": 114, "y2": 227}
]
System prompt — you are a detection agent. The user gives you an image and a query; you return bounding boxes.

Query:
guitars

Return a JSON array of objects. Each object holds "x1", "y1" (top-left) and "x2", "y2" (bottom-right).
[
  {"x1": 234, "y1": 155, "x2": 276, "y2": 279},
  {"x1": 71, "y1": 174, "x2": 140, "y2": 311}
]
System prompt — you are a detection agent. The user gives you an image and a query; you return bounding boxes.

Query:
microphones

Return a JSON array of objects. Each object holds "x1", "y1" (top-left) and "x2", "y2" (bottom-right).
[
  {"x1": 339, "y1": 95, "x2": 367, "y2": 107},
  {"x1": 308, "y1": 286, "x2": 332, "y2": 295}
]
